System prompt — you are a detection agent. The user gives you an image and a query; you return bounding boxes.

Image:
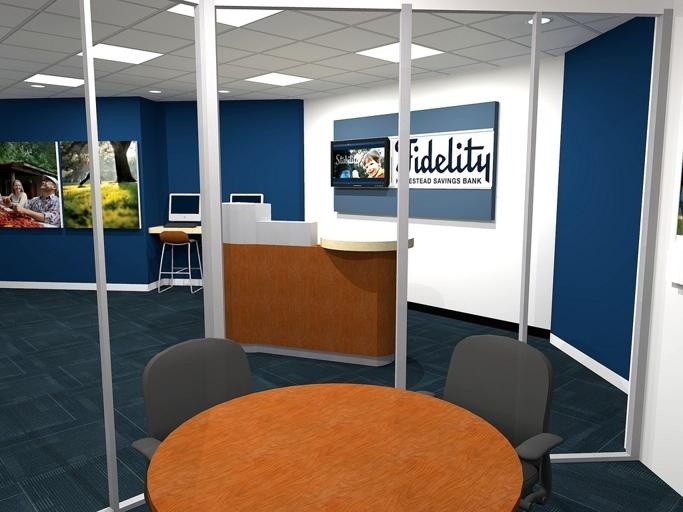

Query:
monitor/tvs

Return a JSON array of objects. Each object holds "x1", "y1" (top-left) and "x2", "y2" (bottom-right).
[
  {"x1": 169, "y1": 193, "x2": 201, "y2": 221},
  {"x1": 331, "y1": 137, "x2": 390, "y2": 187},
  {"x1": 230, "y1": 193, "x2": 264, "y2": 204}
]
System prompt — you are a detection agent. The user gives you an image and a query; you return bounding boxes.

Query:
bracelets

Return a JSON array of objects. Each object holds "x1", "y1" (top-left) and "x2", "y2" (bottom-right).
[{"x1": 9, "y1": 204, "x2": 14, "y2": 209}]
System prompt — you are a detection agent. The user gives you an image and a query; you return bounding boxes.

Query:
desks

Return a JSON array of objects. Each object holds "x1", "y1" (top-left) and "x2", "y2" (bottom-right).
[
  {"x1": 144, "y1": 381, "x2": 527, "y2": 512},
  {"x1": 148, "y1": 224, "x2": 204, "y2": 235}
]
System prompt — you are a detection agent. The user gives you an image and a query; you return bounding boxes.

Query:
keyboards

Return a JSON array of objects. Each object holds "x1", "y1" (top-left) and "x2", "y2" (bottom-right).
[{"x1": 164, "y1": 222, "x2": 197, "y2": 228}]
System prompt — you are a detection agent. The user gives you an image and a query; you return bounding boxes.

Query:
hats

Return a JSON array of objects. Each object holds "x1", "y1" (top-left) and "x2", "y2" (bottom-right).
[{"x1": 42, "y1": 175, "x2": 58, "y2": 193}]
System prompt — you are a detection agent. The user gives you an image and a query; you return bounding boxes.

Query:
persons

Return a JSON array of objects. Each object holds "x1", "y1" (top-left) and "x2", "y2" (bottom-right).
[
  {"x1": 9, "y1": 179, "x2": 28, "y2": 208},
  {"x1": 361, "y1": 150, "x2": 384, "y2": 179},
  {"x1": 2, "y1": 175, "x2": 60, "y2": 228}
]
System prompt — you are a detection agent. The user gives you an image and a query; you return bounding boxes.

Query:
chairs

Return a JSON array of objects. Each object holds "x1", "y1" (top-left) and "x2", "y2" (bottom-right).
[
  {"x1": 415, "y1": 334, "x2": 564, "y2": 512},
  {"x1": 132, "y1": 336, "x2": 256, "y2": 463},
  {"x1": 158, "y1": 231, "x2": 204, "y2": 295}
]
